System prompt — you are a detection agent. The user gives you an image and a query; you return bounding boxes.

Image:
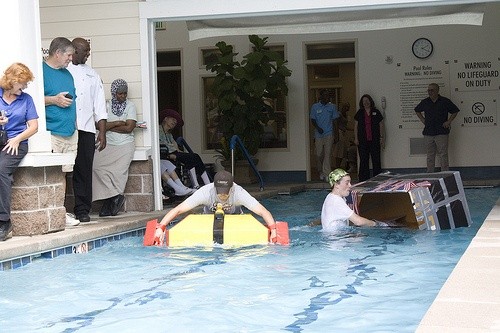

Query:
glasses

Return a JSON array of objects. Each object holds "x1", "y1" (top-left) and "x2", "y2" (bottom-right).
[{"x1": 427, "y1": 89, "x2": 434, "y2": 92}]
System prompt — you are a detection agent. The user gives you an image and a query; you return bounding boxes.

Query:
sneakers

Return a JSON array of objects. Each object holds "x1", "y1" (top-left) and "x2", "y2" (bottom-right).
[
  {"x1": 66, "y1": 212, "x2": 80, "y2": 225},
  {"x1": 0, "y1": 219, "x2": 13, "y2": 241},
  {"x1": 182, "y1": 186, "x2": 197, "y2": 194},
  {"x1": 175, "y1": 187, "x2": 193, "y2": 196}
]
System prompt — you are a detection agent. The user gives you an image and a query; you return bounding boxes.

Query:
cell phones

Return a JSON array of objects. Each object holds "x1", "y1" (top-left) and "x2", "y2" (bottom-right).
[{"x1": 65, "y1": 93, "x2": 73, "y2": 99}]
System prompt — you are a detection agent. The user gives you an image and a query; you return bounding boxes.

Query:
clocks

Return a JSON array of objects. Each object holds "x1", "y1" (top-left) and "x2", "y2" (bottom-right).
[{"x1": 412, "y1": 37, "x2": 433, "y2": 59}]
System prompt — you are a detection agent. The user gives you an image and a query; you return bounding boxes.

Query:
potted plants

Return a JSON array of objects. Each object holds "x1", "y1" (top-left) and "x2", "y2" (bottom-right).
[{"x1": 202, "y1": 34, "x2": 293, "y2": 184}]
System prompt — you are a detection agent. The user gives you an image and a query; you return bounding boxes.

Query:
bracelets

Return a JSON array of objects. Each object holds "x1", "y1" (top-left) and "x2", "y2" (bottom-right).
[{"x1": 380, "y1": 137, "x2": 383, "y2": 138}]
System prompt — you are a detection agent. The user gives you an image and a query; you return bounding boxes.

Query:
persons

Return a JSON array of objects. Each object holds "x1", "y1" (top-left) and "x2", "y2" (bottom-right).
[
  {"x1": 159, "y1": 108, "x2": 209, "y2": 190},
  {"x1": 43, "y1": 37, "x2": 80, "y2": 226},
  {"x1": 0, "y1": 62, "x2": 39, "y2": 242},
  {"x1": 161, "y1": 182, "x2": 170, "y2": 200},
  {"x1": 155, "y1": 171, "x2": 277, "y2": 246},
  {"x1": 310, "y1": 89, "x2": 339, "y2": 182},
  {"x1": 336, "y1": 101, "x2": 350, "y2": 168},
  {"x1": 321, "y1": 168, "x2": 385, "y2": 239},
  {"x1": 160, "y1": 160, "x2": 197, "y2": 195},
  {"x1": 92, "y1": 79, "x2": 137, "y2": 216},
  {"x1": 65, "y1": 38, "x2": 108, "y2": 222},
  {"x1": 354, "y1": 94, "x2": 385, "y2": 182},
  {"x1": 414, "y1": 83, "x2": 460, "y2": 173}
]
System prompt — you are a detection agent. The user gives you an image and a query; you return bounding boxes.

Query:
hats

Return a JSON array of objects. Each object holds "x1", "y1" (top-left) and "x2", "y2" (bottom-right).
[
  {"x1": 160, "y1": 109, "x2": 184, "y2": 127},
  {"x1": 328, "y1": 169, "x2": 349, "y2": 187},
  {"x1": 214, "y1": 171, "x2": 233, "y2": 194}
]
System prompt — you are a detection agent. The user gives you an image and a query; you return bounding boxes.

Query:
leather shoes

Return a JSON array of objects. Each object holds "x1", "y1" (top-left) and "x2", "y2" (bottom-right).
[
  {"x1": 78, "y1": 215, "x2": 90, "y2": 222},
  {"x1": 99, "y1": 198, "x2": 112, "y2": 217},
  {"x1": 110, "y1": 194, "x2": 126, "y2": 216}
]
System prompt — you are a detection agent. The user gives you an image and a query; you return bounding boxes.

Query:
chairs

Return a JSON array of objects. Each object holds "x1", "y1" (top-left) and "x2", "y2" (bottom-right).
[
  {"x1": 159, "y1": 144, "x2": 198, "y2": 207},
  {"x1": 261, "y1": 125, "x2": 274, "y2": 147}
]
